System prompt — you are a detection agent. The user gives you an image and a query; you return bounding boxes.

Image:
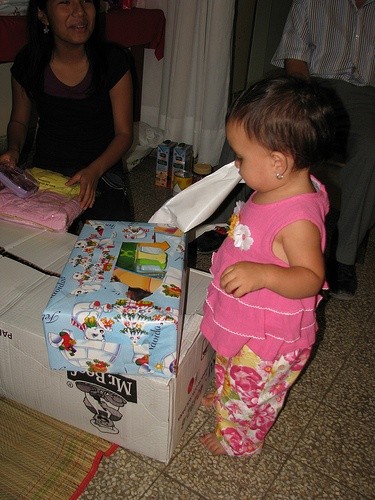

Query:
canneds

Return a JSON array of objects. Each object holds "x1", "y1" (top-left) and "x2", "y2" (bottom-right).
[
  {"x1": 172, "y1": 170, "x2": 193, "y2": 196},
  {"x1": 192, "y1": 163, "x2": 211, "y2": 186}
]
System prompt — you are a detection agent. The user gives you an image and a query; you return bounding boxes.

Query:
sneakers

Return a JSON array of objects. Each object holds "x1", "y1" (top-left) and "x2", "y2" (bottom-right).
[
  {"x1": 193, "y1": 230, "x2": 226, "y2": 254},
  {"x1": 326, "y1": 261, "x2": 357, "y2": 300}
]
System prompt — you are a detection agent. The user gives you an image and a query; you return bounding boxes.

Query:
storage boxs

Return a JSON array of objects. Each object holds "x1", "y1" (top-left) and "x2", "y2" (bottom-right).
[
  {"x1": 0, "y1": 219, "x2": 215, "y2": 466},
  {"x1": 42, "y1": 219, "x2": 187, "y2": 379}
]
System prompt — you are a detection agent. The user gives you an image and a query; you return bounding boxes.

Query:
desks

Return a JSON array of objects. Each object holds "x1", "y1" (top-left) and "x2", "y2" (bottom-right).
[{"x1": 0, "y1": 6, "x2": 166, "y2": 122}]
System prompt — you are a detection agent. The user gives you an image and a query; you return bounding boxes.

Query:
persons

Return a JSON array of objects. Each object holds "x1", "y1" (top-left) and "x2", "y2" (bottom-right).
[
  {"x1": 272, "y1": 0, "x2": 375, "y2": 301},
  {"x1": 199, "y1": 74, "x2": 328, "y2": 456},
  {"x1": 0, "y1": 0, "x2": 134, "y2": 236}
]
